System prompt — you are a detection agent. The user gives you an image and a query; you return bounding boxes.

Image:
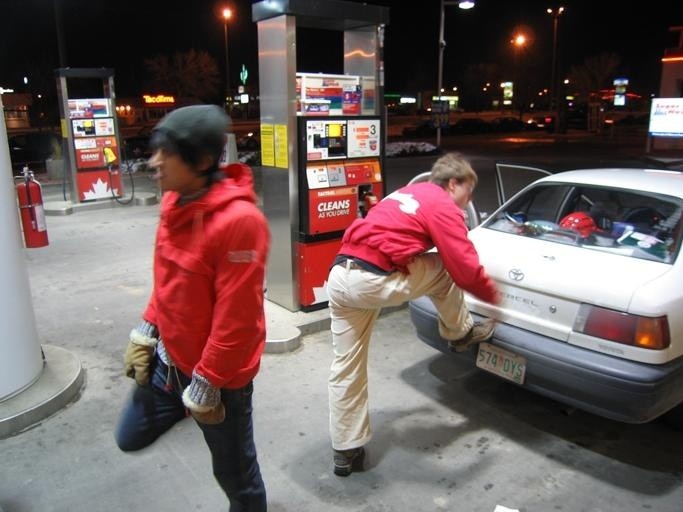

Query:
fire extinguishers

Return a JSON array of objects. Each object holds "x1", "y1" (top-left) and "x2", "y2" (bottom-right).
[{"x1": 14, "y1": 166, "x2": 49, "y2": 248}]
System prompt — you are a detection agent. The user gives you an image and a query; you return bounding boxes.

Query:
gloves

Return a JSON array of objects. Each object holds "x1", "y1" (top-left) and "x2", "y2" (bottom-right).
[
  {"x1": 183, "y1": 388, "x2": 226, "y2": 425},
  {"x1": 124, "y1": 331, "x2": 155, "y2": 387}
]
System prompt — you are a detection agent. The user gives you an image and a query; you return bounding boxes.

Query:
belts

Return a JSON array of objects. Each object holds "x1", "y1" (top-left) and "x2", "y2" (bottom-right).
[{"x1": 338, "y1": 260, "x2": 361, "y2": 270}]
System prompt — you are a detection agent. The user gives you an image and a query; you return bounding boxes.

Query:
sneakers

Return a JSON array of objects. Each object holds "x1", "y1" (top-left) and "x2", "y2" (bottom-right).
[
  {"x1": 332, "y1": 444, "x2": 365, "y2": 477},
  {"x1": 447, "y1": 317, "x2": 496, "y2": 353}
]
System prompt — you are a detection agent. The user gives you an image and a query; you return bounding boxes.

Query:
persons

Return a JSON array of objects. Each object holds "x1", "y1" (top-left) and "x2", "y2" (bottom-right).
[
  {"x1": 116, "y1": 104, "x2": 269, "y2": 509},
  {"x1": 325, "y1": 151, "x2": 501, "y2": 477}
]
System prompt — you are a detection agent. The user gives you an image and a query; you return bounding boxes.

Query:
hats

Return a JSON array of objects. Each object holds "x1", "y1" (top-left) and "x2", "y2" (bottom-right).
[{"x1": 151, "y1": 104, "x2": 232, "y2": 154}]
